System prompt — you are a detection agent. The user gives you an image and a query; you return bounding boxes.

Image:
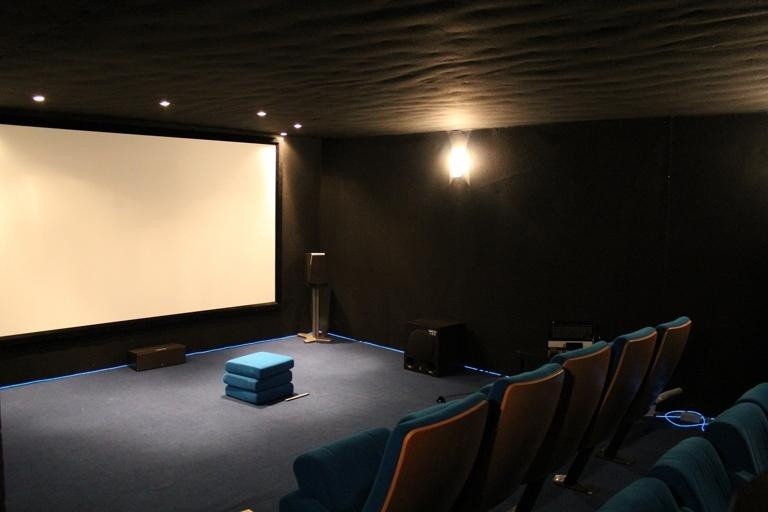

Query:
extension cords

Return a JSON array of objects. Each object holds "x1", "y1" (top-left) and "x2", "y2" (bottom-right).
[{"x1": 680, "y1": 412, "x2": 702, "y2": 425}]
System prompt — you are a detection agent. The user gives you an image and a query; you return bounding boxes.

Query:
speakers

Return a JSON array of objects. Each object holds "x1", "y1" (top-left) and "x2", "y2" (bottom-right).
[
  {"x1": 303, "y1": 247, "x2": 329, "y2": 288},
  {"x1": 126, "y1": 342, "x2": 188, "y2": 371},
  {"x1": 403, "y1": 316, "x2": 463, "y2": 378}
]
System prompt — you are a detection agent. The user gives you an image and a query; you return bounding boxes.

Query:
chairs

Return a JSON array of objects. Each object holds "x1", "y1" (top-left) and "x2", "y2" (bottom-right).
[
  {"x1": 563, "y1": 325, "x2": 659, "y2": 493},
  {"x1": 517, "y1": 340, "x2": 614, "y2": 509},
  {"x1": 593, "y1": 475, "x2": 696, "y2": 512},
  {"x1": 701, "y1": 402, "x2": 765, "y2": 478},
  {"x1": 609, "y1": 316, "x2": 695, "y2": 454},
  {"x1": 477, "y1": 360, "x2": 568, "y2": 509},
  {"x1": 278, "y1": 388, "x2": 491, "y2": 512},
  {"x1": 643, "y1": 434, "x2": 758, "y2": 508},
  {"x1": 735, "y1": 381, "x2": 766, "y2": 410}
]
